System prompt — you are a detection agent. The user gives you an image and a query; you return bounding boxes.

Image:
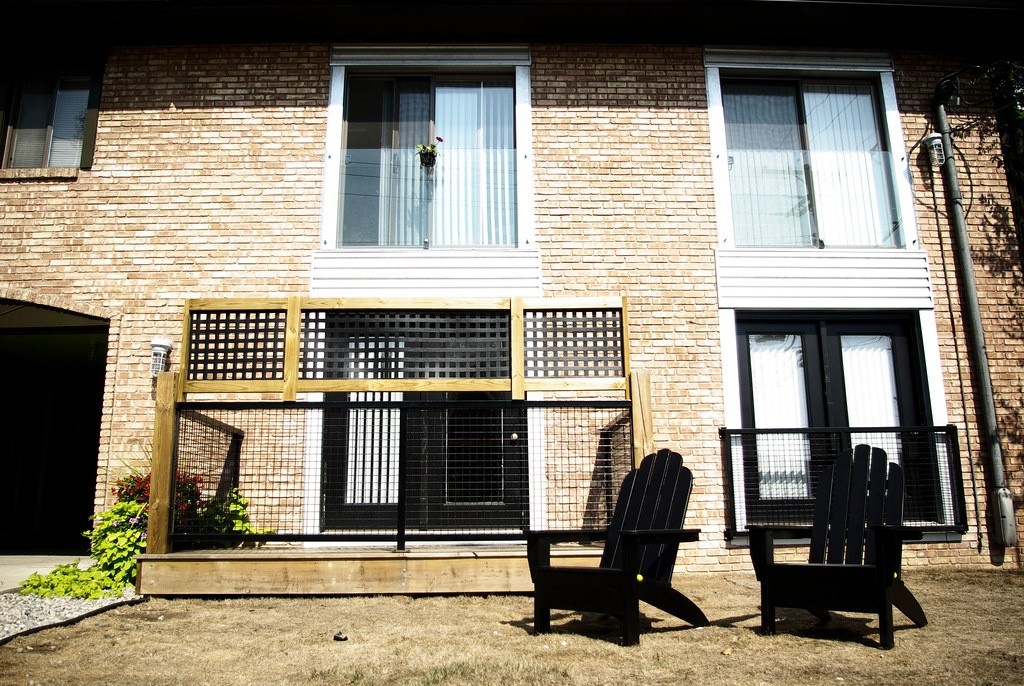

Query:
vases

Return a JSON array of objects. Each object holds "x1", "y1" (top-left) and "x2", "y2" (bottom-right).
[{"x1": 420, "y1": 153, "x2": 436, "y2": 168}]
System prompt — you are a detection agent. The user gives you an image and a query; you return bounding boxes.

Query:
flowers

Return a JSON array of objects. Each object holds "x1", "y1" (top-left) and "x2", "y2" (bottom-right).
[{"x1": 415, "y1": 136, "x2": 444, "y2": 155}]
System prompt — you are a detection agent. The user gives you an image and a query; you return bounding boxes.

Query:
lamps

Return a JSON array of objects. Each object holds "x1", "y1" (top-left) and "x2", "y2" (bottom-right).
[
  {"x1": 150, "y1": 338, "x2": 173, "y2": 378},
  {"x1": 923, "y1": 133, "x2": 946, "y2": 166}
]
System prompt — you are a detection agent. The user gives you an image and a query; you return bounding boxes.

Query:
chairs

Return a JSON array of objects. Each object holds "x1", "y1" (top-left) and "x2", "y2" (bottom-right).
[
  {"x1": 745, "y1": 443, "x2": 928, "y2": 651},
  {"x1": 523, "y1": 449, "x2": 710, "y2": 647}
]
